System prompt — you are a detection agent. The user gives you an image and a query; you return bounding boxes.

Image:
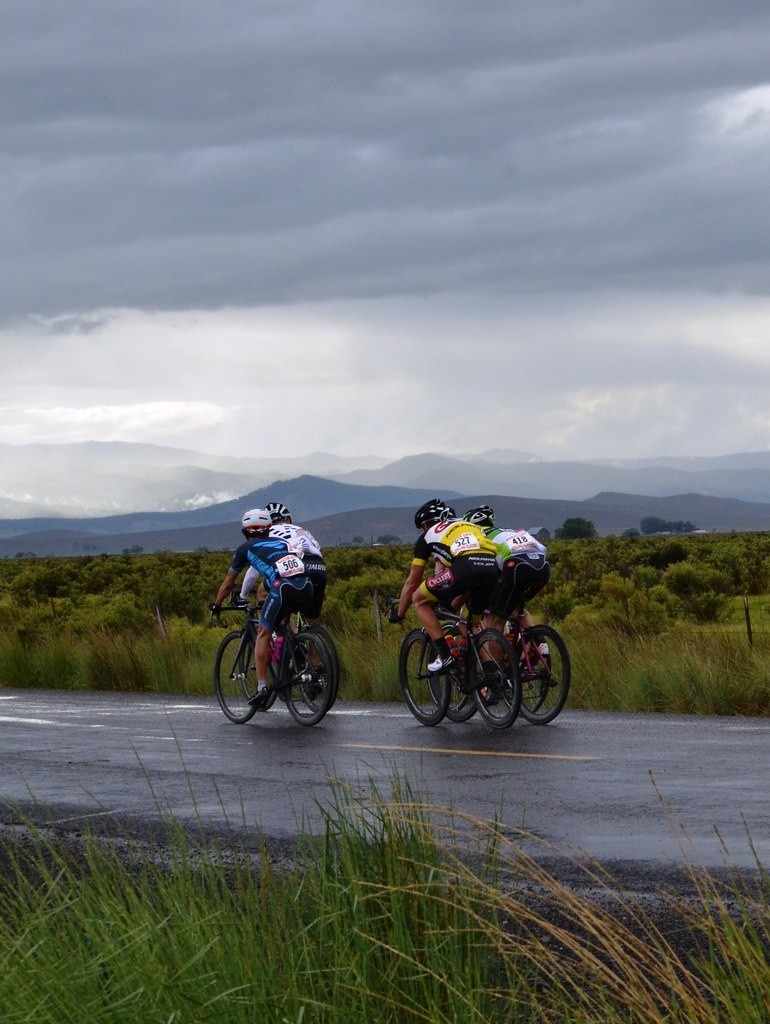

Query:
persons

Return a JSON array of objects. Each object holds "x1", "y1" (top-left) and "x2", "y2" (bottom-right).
[
  {"x1": 210, "y1": 508, "x2": 313, "y2": 705},
  {"x1": 463, "y1": 504, "x2": 551, "y2": 706},
  {"x1": 389, "y1": 498, "x2": 507, "y2": 701},
  {"x1": 238, "y1": 502, "x2": 328, "y2": 701}
]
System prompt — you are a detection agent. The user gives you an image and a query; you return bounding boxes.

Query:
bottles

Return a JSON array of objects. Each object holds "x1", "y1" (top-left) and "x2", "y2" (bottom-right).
[
  {"x1": 267, "y1": 635, "x2": 284, "y2": 662},
  {"x1": 444, "y1": 634, "x2": 458, "y2": 658},
  {"x1": 456, "y1": 634, "x2": 468, "y2": 661},
  {"x1": 503, "y1": 627, "x2": 517, "y2": 644}
]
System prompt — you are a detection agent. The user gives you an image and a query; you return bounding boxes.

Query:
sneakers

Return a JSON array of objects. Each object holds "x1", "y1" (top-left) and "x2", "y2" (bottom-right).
[
  {"x1": 427, "y1": 654, "x2": 457, "y2": 672},
  {"x1": 248, "y1": 687, "x2": 269, "y2": 706},
  {"x1": 480, "y1": 686, "x2": 495, "y2": 702}
]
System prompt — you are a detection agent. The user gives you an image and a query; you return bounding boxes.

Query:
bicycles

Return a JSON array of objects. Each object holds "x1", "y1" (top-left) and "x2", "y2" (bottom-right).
[
  {"x1": 427, "y1": 582, "x2": 570, "y2": 725},
  {"x1": 208, "y1": 588, "x2": 339, "y2": 726},
  {"x1": 383, "y1": 594, "x2": 522, "y2": 728}
]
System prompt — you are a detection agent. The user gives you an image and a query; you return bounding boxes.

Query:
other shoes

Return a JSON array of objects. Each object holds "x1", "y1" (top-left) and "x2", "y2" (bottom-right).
[{"x1": 529, "y1": 642, "x2": 549, "y2": 656}]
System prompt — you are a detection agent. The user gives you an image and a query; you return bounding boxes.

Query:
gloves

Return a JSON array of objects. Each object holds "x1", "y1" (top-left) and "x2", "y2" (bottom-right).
[
  {"x1": 208, "y1": 602, "x2": 220, "y2": 617},
  {"x1": 389, "y1": 608, "x2": 406, "y2": 624},
  {"x1": 231, "y1": 591, "x2": 247, "y2": 607}
]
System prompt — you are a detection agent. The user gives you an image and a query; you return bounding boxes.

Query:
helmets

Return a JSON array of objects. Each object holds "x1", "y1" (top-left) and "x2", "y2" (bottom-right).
[
  {"x1": 242, "y1": 508, "x2": 272, "y2": 532},
  {"x1": 415, "y1": 499, "x2": 456, "y2": 527},
  {"x1": 263, "y1": 502, "x2": 293, "y2": 524},
  {"x1": 463, "y1": 505, "x2": 495, "y2": 530}
]
